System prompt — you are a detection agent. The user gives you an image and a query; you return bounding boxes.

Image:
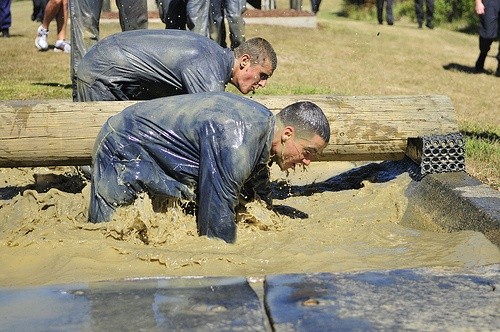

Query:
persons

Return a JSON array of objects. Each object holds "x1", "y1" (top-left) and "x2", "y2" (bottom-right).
[
  {"x1": 158, "y1": 0, "x2": 248, "y2": 52},
  {"x1": 376, "y1": 0, "x2": 394, "y2": 25},
  {"x1": 69, "y1": 0, "x2": 149, "y2": 102},
  {"x1": 474, "y1": 0, "x2": 500, "y2": 77},
  {"x1": 414, "y1": 0, "x2": 435, "y2": 29},
  {"x1": 77, "y1": 29, "x2": 278, "y2": 180},
  {"x1": 88, "y1": 92, "x2": 331, "y2": 242},
  {"x1": 35, "y1": 0, "x2": 71, "y2": 52},
  {"x1": 0, "y1": 0, "x2": 12, "y2": 37},
  {"x1": 31, "y1": 0, "x2": 47, "y2": 22}
]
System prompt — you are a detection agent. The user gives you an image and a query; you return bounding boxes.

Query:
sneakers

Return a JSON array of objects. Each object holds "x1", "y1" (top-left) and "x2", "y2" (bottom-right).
[
  {"x1": 54, "y1": 40, "x2": 72, "y2": 53},
  {"x1": 35, "y1": 25, "x2": 49, "y2": 50}
]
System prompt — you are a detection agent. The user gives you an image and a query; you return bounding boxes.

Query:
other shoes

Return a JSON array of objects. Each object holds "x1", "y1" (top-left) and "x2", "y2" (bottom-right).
[
  {"x1": 3, "y1": 30, "x2": 10, "y2": 38},
  {"x1": 32, "y1": 11, "x2": 41, "y2": 20}
]
